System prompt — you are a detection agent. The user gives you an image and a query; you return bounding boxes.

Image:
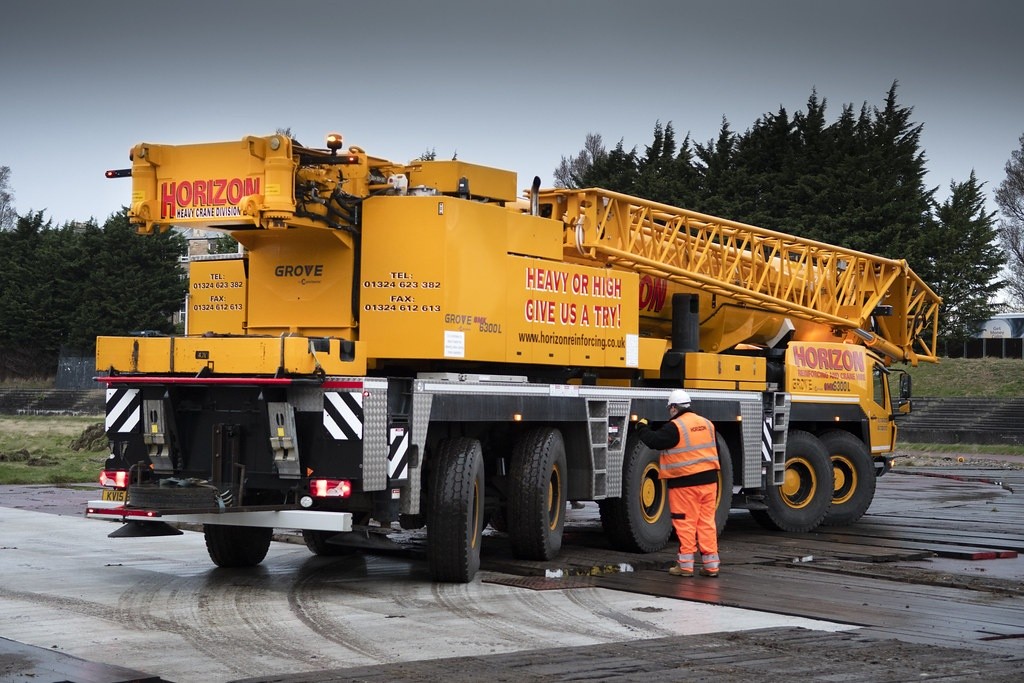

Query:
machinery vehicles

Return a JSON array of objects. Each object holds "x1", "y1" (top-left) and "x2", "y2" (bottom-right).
[{"x1": 85, "y1": 130, "x2": 946, "y2": 586}]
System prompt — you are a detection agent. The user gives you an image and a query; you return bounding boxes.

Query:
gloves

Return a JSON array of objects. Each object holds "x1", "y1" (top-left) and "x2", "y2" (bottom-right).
[{"x1": 635, "y1": 418, "x2": 649, "y2": 430}]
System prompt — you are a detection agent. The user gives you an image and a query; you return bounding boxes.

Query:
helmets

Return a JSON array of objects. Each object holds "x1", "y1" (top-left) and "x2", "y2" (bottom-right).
[{"x1": 668, "y1": 389, "x2": 692, "y2": 405}]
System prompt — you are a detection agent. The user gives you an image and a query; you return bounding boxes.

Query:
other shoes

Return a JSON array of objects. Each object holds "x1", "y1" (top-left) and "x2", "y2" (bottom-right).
[
  {"x1": 670, "y1": 565, "x2": 694, "y2": 576},
  {"x1": 700, "y1": 570, "x2": 717, "y2": 576}
]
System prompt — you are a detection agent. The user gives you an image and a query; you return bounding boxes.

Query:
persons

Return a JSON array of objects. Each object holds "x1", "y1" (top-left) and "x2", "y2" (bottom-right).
[{"x1": 636, "y1": 389, "x2": 721, "y2": 578}]
